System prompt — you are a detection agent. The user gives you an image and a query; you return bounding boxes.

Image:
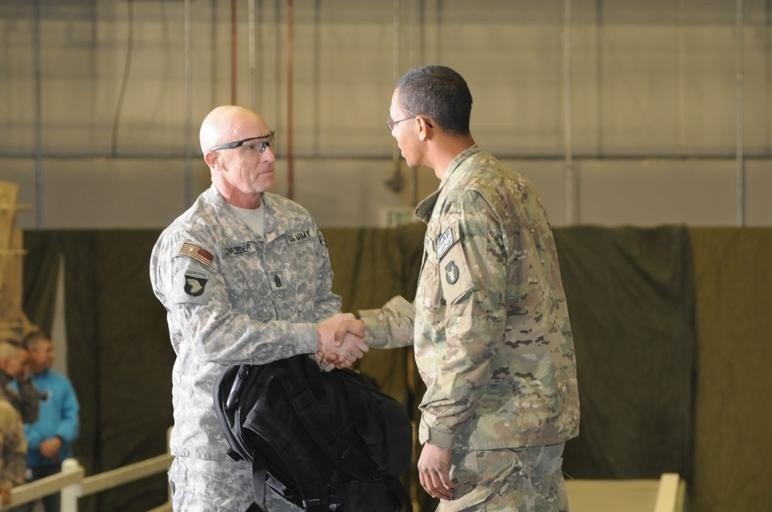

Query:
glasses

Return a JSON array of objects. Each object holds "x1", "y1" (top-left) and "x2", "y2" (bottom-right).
[
  {"x1": 387, "y1": 117, "x2": 433, "y2": 130},
  {"x1": 208, "y1": 130, "x2": 275, "y2": 153}
]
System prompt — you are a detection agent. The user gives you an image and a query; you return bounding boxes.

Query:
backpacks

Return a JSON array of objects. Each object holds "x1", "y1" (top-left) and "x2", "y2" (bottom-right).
[{"x1": 213, "y1": 353, "x2": 412, "y2": 512}]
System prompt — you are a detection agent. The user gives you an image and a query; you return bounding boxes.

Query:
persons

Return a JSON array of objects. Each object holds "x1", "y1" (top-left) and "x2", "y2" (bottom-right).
[
  {"x1": 149, "y1": 106, "x2": 369, "y2": 510},
  {"x1": 315, "y1": 66, "x2": 582, "y2": 512},
  {"x1": 0, "y1": 330, "x2": 81, "y2": 512}
]
